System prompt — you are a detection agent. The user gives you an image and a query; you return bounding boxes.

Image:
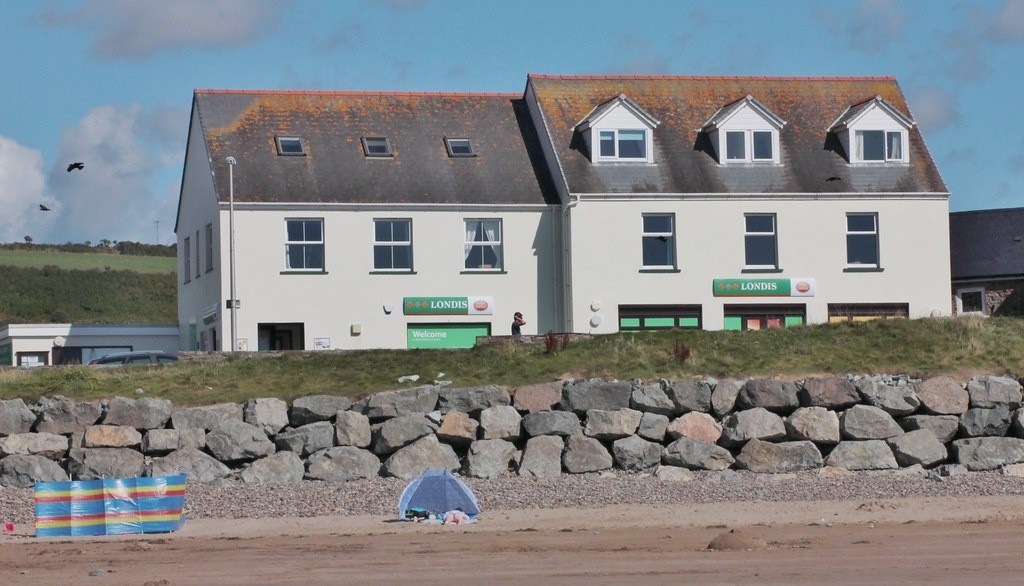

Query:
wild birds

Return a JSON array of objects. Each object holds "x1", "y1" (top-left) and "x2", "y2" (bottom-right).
[
  {"x1": 67, "y1": 163, "x2": 84, "y2": 172},
  {"x1": 40, "y1": 204, "x2": 51, "y2": 211}
]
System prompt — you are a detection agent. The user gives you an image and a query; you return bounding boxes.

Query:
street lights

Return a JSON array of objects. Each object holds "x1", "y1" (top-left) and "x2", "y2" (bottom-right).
[{"x1": 223, "y1": 156, "x2": 239, "y2": 353}]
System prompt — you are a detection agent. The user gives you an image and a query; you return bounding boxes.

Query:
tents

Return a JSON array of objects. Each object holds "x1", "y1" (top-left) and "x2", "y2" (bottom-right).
[{"x1": 398, "y1": 468, "x2": 478, "y2": 519}]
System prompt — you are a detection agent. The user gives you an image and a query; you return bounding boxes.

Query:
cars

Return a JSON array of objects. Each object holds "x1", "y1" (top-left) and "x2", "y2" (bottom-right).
[{"x1": 86, "y1": 350, "x2": 180, "y2": 366}]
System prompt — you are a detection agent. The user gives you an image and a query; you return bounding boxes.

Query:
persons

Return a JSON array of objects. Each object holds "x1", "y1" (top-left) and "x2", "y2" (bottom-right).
[
  {"x1": 444, "y1": 507, "x2": 470, "y2": 526},
  {"x1": 511, "y1": 312, "x2": 526, "y2": 335}
]
